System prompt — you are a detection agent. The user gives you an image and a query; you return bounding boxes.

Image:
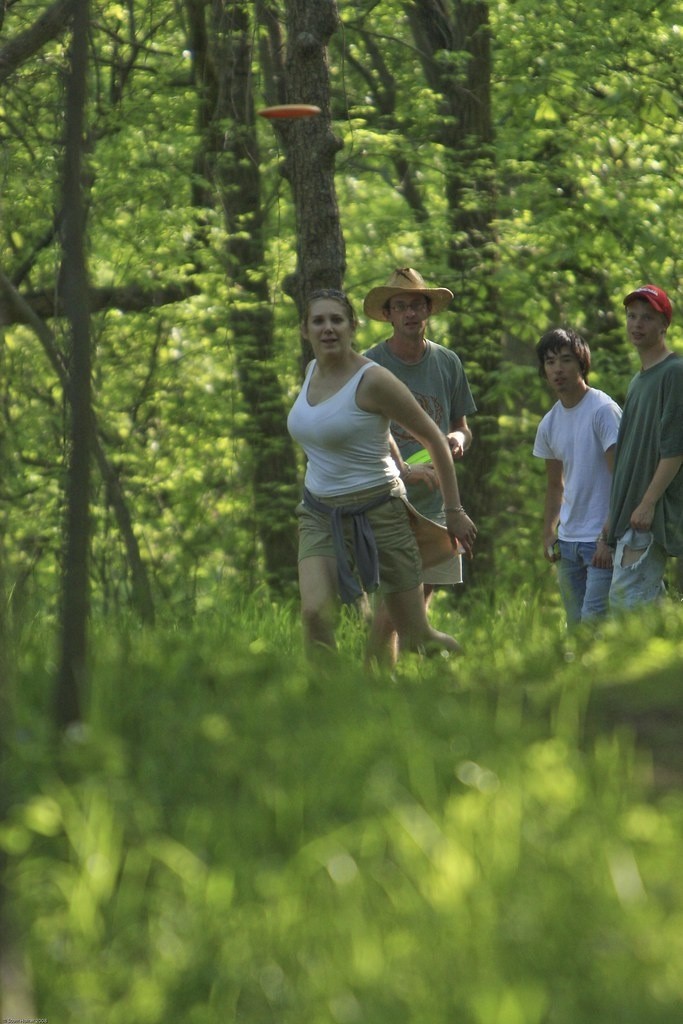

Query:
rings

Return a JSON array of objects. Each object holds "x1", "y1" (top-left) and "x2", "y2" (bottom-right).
[{"x1": 400, "y1": 462, "x2": 412, "y2": 481}]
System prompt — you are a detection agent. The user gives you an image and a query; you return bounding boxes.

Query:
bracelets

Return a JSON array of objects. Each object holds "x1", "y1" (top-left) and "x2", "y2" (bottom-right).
[{"x1": 443, "y1": 505, "x2": 466, "y2": 513}]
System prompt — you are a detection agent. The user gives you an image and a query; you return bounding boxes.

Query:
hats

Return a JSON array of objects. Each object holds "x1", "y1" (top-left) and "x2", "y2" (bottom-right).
[
  {"x1": 364, "y1": 267, "x2": 455, "y2": 322},
  {"x1": 623, "y1": 285, "x2": 672, "y2": 323}
]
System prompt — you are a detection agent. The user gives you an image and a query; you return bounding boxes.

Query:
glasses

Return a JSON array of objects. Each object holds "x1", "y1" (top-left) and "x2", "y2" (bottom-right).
[{"x1": 388, "y1": 302, "x2": 427, "y2": 312}]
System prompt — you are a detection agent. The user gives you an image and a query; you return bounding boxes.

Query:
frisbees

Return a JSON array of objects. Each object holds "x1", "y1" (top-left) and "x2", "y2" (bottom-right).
[{"x1": 256, "y1": 104, "x2": 321, "y2": 118}]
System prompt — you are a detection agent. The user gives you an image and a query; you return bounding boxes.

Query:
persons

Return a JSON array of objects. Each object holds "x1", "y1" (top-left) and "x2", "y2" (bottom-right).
[
  {"x1": 283, "y1": 287, "x2": 478, "y2": 670},
  {"x1": 358, "y1": 267, "x2": 479, "y2": 677},
  {"x1": 530, "y1": 328, "x2": 623, "y2": 633},
  {"x1": 602, "y1": 284, "x2": 683, "y2": 626}
]
